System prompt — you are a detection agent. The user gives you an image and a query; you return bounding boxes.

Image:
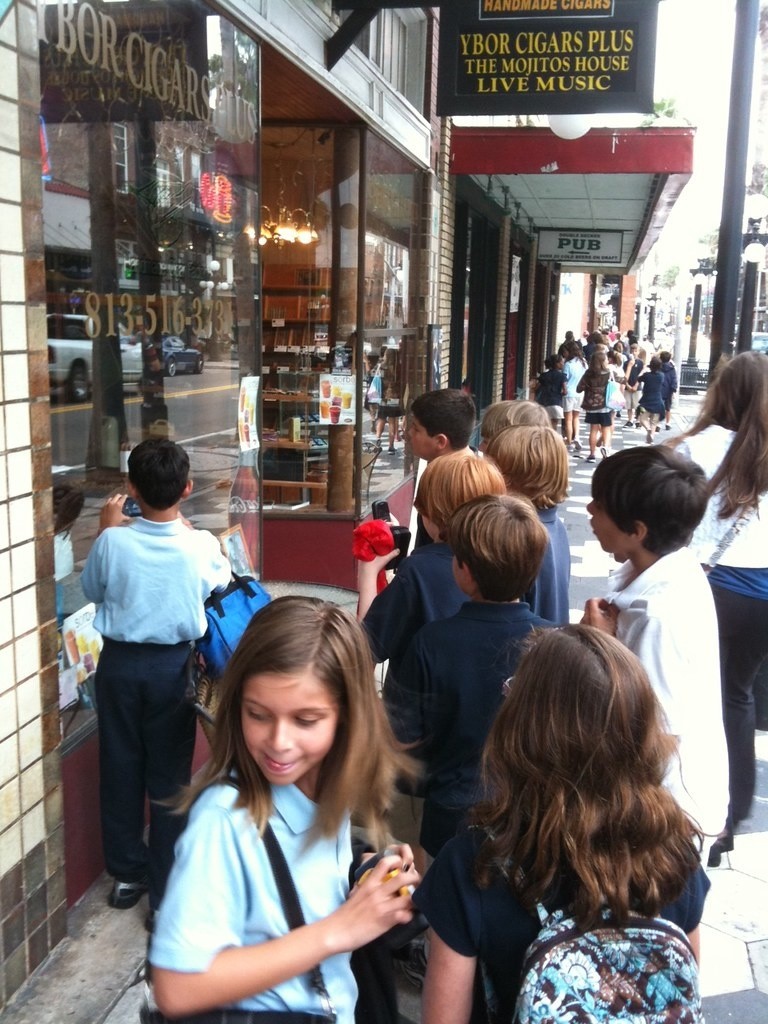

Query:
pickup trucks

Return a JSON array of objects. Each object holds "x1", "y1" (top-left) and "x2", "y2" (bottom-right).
[{"x1": 47, "y1": 314, "x2": 143, "y2": 402}]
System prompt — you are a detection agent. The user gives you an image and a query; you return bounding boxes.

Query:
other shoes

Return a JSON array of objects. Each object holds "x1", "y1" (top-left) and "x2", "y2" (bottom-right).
[
  {"x1": 144, "y1": 904, "x2": 159, "y2": 931},
  {"x1": 600, "y1": 447, "x2": 610, "y2": 460},
  {"x1": 655, "y1": 426, "x2": 660, "y2": 432},
  {"x1": 635, "y1": 422, "x2": 642, "y2": 428},
  {"x1": 646, "y1": 430, "x2": 653, "y2": 443},
  {"x1": 567, "y1": 445, "x2": 574, "y2": 452},
  {"x1": 574, "y1": 437, "x2": 582, "y2": 449},
  {"x1": 586, "y1": 455, "x2": 596, "y2": 462},
  {"x1": 108, "y1": 872, "x2": 151, "y2": 908},
  {"x1": 665, "y1": 424, "x2": 671, "y2": 431},
  {"x1": 623, "y1": 421, "x2": 633, "y2": 427},
  {"x1": 388, "y1": 448, "x2": 397, "y2": 454},
  {"x1": 616, "y1": 414, "x2": 621, "y2": 417}
]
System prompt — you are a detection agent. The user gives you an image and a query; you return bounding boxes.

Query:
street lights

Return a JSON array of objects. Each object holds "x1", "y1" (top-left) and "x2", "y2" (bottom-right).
[
  {"x1": 736, "y1": 191, "x2": 768, "y2": 353},
  {"x1": 679, "y1": 254, "x2": 714, "y2": 395},
  {"x1": 645, "y1": 285, "x2": 663, "y2": 340}
]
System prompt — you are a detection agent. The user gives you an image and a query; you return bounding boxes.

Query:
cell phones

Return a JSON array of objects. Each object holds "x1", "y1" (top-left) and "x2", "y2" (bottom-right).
[
  {"x1": 122, "y1": 495, "x2": 142, "y2": 516},
  {"x1": 372, "y1": 500, "x2": 391, "y2": 523}
]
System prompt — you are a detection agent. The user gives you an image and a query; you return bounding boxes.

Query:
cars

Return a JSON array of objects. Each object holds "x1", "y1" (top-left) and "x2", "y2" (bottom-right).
[
  {"x1": 161, "y1": 333, "x2": 206, "y2": 376},
  {"x1": 751, "y1": 331, "x2": 768, "y2": 353}
]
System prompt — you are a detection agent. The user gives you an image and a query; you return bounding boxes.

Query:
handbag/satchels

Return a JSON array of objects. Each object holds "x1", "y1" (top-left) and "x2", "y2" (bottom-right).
[
  {"x1": 194, "y1": 570, "x2": 273, "y2": 679},
  {"x1": 605, "y1": 368, "x2": 625, "y2": 411},
  {"x1": 140, "y1": 1005, "x2": 338, "y2": 1024},
  {"x1": 367, "y1": 364, "x2": 383, "y2": 404}
]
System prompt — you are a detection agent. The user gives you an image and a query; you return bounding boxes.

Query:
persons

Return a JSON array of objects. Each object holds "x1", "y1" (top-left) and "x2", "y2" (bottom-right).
[
  {"x1": 673, "y1": 351, "x2": 768, "y2": 823},
  {"x1": 147, "y1": 596, "x2": 420, "y2": 1024},
  {"x1": 80, "y1": 438, "x2": 231, "y2": 909},
  {"x1": 359, "y1": 389, "x2": 570, "y2": 671},
  {"x1": 363, "y1": 340, "x2": 401, "y2": 454},
  {"x1": 578, "y1": 444, "x2": 730, "y2": 864},
  {"x1": 392, "y1": 495, "x2": 561, "y2": 856},
  {"x1": 536, "y1": 325, "x2": 677, "y2": 463},
  {"x1": 415, "y1": 624, "x2": 710, "y2": 1024}
]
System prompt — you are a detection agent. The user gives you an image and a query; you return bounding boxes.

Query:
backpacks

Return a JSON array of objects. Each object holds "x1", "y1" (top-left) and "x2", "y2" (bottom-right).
[{"x1": 472, "y1": 824, "x2": 704, "y2": 1024}]
